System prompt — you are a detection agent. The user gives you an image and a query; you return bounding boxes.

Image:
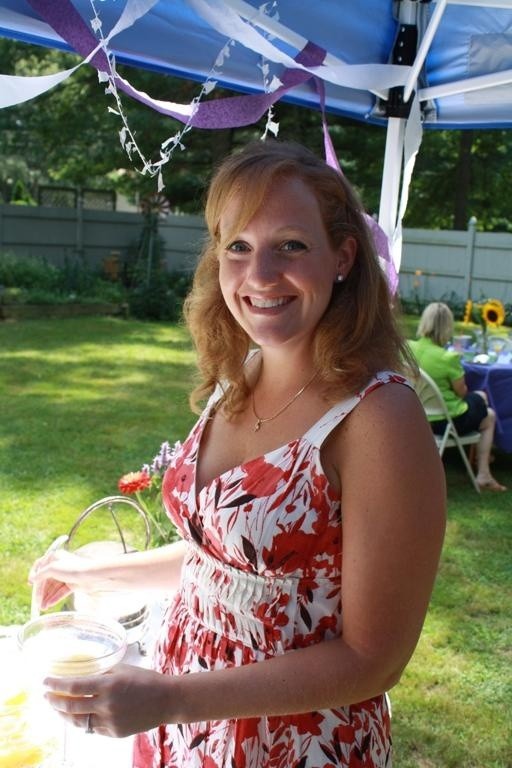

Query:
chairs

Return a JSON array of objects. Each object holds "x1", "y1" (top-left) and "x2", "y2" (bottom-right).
[{"x1": 401, "y1": 360, "x2": 484, "y2": 495}]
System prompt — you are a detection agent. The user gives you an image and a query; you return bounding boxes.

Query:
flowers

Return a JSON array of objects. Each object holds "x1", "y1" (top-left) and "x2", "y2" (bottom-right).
[{"x1": 461, "y1": 296, "x2": 505, "y2": 332}]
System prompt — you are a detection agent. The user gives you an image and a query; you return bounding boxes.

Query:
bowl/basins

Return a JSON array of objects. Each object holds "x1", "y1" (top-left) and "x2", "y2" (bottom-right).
[{"x1": 452, "y1": 334, "x2": 500, "y2": 367}]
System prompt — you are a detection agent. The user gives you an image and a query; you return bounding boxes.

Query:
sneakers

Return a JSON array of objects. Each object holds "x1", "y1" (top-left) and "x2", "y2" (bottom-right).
[{"x1": 475, "y1": 472, "x2": 508, "y2": 491}]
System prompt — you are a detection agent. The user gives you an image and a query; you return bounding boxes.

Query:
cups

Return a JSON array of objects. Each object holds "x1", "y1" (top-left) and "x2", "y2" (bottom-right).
[{"x1": 17, "y1": 610, "x2": 130, "y2": 675}]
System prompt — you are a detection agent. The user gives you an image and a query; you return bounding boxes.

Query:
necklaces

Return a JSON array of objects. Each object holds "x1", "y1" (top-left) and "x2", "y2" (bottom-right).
[{"x1": 251, "y1": 365, "x2": 322, "y2": 432}]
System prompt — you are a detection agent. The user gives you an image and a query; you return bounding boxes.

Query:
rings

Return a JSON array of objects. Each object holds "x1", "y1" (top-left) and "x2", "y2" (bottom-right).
[{"x1": 84, "y1": 713, "x2": 95, "y2": 734}]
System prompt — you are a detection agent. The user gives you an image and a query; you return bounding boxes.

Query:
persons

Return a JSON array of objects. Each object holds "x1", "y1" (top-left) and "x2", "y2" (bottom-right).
[
  {"x1": 398, "y1": 302, "x2": 507, "y2": 493},
  {"x1": 29, "y1": 139, "x2": 447, "y2": 768}
]
